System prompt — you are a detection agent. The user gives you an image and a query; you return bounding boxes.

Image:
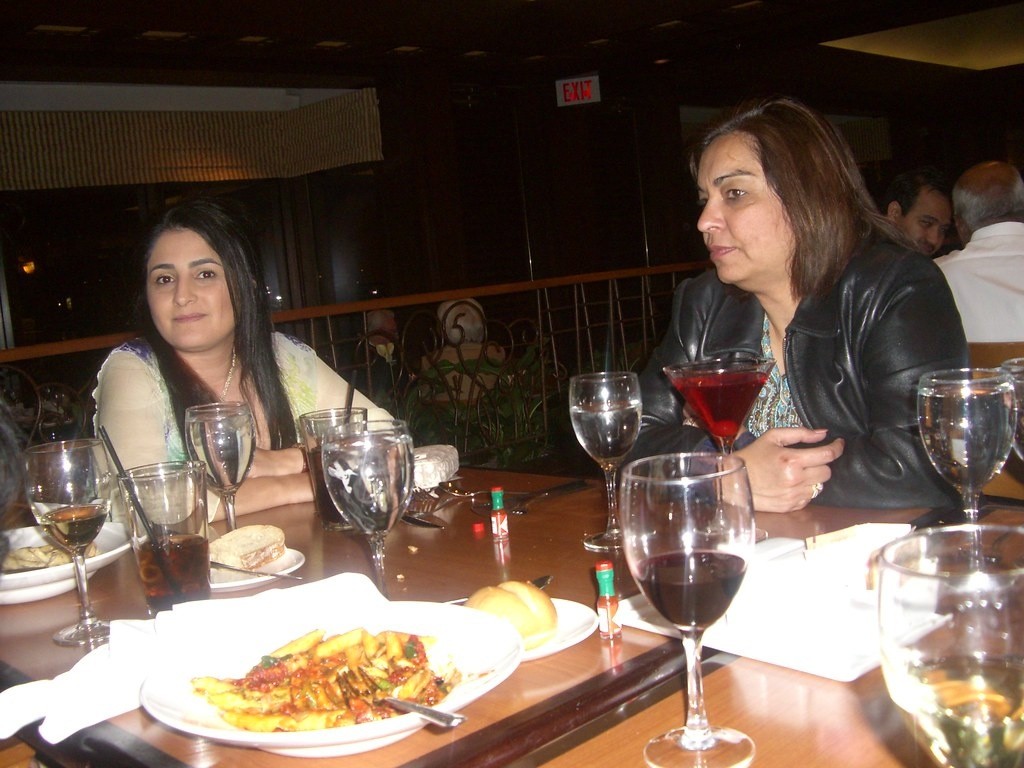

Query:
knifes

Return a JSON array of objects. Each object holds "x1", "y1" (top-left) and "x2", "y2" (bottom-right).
[
  {"x1": 210, "y1": 561, "x2": 302, "y2": 580},
  {"x1": 402, "y1": 515, "x2": 445, "y2": 530}
]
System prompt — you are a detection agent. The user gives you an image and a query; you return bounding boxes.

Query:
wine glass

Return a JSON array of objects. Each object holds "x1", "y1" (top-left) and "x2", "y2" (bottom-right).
[
  {"x1": 569, "y1": 370, "x2": 655, "y2": 551},
  {"x1": 916, "y1": 367, "x2": 1017, "y2": 573},
  {"x1": 617, "y1": 450, "x2": 756, "y2": 768},
  {"x1": 184, "y1": 401, "x2": 256, "y2": 530},
  {"x1": 321, "y1": 418, "x2": 414, "y2": 599},
  {"x1": 663, "y1": 357, "x2": 777, "y2": 543},
  {"x1": 23, "y1": 439, "x2": 113, "y2": 645}
]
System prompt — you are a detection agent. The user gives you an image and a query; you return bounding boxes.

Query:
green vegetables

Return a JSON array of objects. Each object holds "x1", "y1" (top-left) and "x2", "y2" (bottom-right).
[{"x1": 254, "y1": 642, "x2": 449, "y2": 695}]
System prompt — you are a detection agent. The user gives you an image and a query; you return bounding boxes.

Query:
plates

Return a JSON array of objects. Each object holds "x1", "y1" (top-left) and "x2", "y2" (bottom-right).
[
  {"x1": 139, "y1": 601, "x2": 523, "y2": 759},
  {"x1": 0, "y1": 522, "x2": 148, "y2": 605},
  {"x1": 210, "y1": 547, "x2": 305, "y2": 593},
  {"x1": 521, "y1": 597, "x2": 599, "y2": 662}
]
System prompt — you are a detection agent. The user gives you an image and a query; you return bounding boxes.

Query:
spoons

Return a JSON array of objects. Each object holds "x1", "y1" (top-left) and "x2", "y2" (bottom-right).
[
  {"x1": 439, "y1": 481, "x2": 530, "y2": 497},
  {"x1": 443, "y1": 575, "x2": 552, "y2": 603}
]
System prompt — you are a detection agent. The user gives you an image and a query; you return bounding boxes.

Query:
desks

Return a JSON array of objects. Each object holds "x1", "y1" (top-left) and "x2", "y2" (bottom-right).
[{"x1": 0, "y1": 457, "x2": 1024, "y2": 767}]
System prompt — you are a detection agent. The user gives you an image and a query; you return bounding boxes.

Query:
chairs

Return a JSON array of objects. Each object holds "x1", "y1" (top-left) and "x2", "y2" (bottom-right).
[{"x1": 964, "y1": 338, "x2": 1024, "y2": 504}]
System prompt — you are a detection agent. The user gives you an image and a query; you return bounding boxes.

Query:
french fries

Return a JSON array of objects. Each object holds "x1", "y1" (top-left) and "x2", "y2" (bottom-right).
[
  {"x1": 192, "y1": 627, "x2": 436, "y2": 730},
  {"x1": 1, "y1": 541, "x2": 96, "y2": 572}
]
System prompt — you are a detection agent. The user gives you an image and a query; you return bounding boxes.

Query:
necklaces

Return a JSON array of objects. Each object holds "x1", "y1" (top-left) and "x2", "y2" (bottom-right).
[{"x1": 219, "y1": 346, "x2": 237, "y2": 402}]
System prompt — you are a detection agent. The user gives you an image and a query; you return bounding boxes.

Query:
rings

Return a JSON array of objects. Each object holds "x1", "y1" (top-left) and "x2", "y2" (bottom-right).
[{"x1": 811, "y1": 483, "x2": 822, "y2": 499}]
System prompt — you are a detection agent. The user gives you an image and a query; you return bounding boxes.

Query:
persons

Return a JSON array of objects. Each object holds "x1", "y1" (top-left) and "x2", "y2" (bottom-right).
[
  {"x1": 420, "y1": 298, "x2": 506, "y2": 402},
  {"x1": 90, "y1": 200, "x2": 395, "y2": 536},
  {"x1": 360, "y1": 308, "x2": 409, "y2": 401},
  {"x1": 880, "y1": 166, "x2": 953, "y2": 257},
  {"x1": 933, "y1": 160, "x2": 1024, "y2": 343},
  {"x1": 619, "y1": 97, "x2": 969, "y2": 513}
]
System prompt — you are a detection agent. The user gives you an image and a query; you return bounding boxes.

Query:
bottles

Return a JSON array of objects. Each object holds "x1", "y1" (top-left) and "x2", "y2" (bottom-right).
[
  {"x1": 595, "y1": 560, "x2": 623, "y2": 640},
  {"x1": 490, "y1": 486, "x2": 509, "y2": 540}
]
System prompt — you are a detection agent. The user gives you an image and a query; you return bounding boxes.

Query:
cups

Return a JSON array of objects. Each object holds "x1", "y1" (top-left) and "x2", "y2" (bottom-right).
[
  {"x1": 1002, "y1": 357, "x2": 1024, "y2": 461},
  {"x1": 299, "y1": 407, "x2": 367, "y2": 533},
  {"x1": 875, "y1": 524, "x2": 1024, "y2": 768},
  {"x1": 117, "y1": 460, "x2": 213, "y2": 610}
]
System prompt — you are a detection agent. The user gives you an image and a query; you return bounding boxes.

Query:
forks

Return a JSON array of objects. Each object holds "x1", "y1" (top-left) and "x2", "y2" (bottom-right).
[
  {"x1": 415, "y1": 486, "x2": 440, "y2": 502},
  {"x1": 335, "y1": 669, "x2": 467, "y2": 727}
]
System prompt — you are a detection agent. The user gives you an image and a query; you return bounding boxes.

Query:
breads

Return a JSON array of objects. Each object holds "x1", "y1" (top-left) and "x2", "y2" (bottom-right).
[
  {"x1": 209, "y1": 524, "x2": 287, "y2": 570},
  {"x1": 461, "y1": 580, "x2": 559, "y2": 651}
]
